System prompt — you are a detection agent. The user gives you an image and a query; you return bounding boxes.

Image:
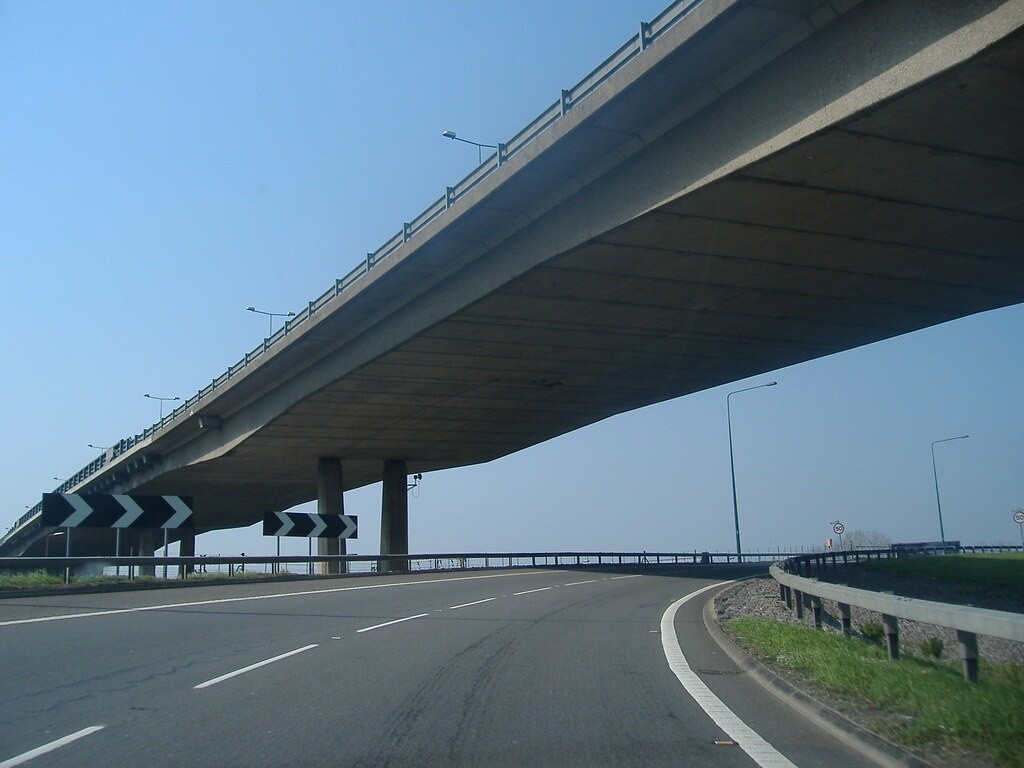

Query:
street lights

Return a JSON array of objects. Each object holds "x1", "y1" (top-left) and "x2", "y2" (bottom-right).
[
  {"x1": 44, "y1": 532, "x2": 65, "y2": 557},
  {"x1": 726, "y1": 380, "x2": 781, "y2": 563},
  {"x1": 929, "y1": 434, "x2": 971, "y2": 555},
  {"x1": 88, "y1": 444, "x2": 110, "y2": 455},
  {"x1": 441, "y1": 129, "x2": 496, "y2": 165},
  {"x1": 245, "y1": 305, "x2": 297, "y2": 337},
  {"x1": 144, "y1": 393, "x2": 181, "y2": 422}
]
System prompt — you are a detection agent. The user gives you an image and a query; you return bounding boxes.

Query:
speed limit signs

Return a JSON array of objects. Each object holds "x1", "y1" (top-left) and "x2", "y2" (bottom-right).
[
  {"x1": 1012, "y1": 510, "x2": 1024, "y2": 524},
  {"x1": 832, "y1": 523, "x2": 845, "y2": 534}
]
